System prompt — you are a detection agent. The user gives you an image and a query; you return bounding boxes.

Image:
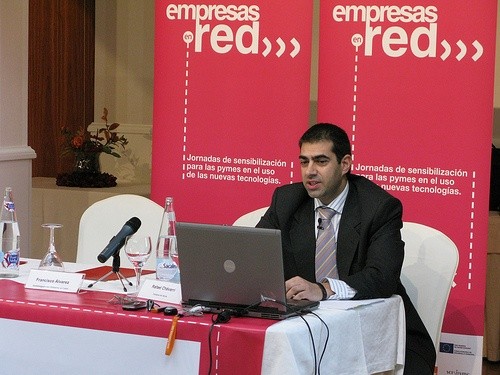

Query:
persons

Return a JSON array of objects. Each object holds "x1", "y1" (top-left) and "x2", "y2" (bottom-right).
[{"x1": 255, "y1": 123, "x2": 436, "y2": 375}]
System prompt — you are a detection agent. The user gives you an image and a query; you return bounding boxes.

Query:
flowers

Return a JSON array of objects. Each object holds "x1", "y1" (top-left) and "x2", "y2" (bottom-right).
[{"x1": 57, "y1": 107, "x2": 128, "y2": 160}]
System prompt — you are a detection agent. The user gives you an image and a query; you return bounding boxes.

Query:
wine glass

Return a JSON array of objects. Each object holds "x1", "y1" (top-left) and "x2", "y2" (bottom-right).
[
  {"x1": 121, "y1": 235, "x2": 152, "y2": 309},
  {"x1": 39, "y1": 223, "x2": 66, "y2": 273}
]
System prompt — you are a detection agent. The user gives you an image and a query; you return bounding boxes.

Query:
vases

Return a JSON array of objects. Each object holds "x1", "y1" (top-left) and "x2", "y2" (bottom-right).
[{"x1": 74, "y1": 152, "x2": 101, "y2": 187}]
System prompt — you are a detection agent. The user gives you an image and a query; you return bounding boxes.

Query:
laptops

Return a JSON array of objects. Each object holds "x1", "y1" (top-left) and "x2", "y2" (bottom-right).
[{"x1": 175, "y1": 222, "x2": 321, "y2": 321}]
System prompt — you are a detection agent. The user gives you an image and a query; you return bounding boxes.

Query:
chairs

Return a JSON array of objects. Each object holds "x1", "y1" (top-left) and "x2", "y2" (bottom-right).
[
  {"x1": 78, "y1": 193, "x2": 169, "y2": 270},
  {"x1": 400, "y1": 219, "x2": 460, "y2": 351}
]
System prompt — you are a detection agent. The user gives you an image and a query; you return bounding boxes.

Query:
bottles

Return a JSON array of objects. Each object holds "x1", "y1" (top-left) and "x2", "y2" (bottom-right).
[
  {"x1": 155, "y1": 196, "x2": 180, "y2": 284},
  {"x1": 0, "y1": 186, "x2": 19, "y2": 278}
]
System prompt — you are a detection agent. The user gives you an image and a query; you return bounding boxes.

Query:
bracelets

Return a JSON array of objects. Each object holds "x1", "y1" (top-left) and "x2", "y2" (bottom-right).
[{"x1": 314, "y1": 282, "x2": 327, "y2": 301}]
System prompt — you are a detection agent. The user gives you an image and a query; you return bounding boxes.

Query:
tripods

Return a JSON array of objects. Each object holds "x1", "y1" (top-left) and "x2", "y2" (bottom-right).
[{"x1": 88, "y1": 247, "x2": 133, "y2": 293}]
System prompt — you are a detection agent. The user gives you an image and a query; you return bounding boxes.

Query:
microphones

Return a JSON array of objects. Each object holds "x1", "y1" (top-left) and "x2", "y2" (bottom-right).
[{"x1": 98, "y1": 217, "x2": 141, "y2": 263}]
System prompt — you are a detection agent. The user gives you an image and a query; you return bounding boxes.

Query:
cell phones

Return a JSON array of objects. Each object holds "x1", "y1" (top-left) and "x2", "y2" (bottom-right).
[{"x1": 123, "y1": 299, "x2": 147, "y2": 309}]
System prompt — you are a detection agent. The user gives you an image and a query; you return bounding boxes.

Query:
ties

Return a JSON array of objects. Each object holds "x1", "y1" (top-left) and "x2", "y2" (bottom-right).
[{"x1": 314, "y1": 207, "x2": 339, "y2": 283}]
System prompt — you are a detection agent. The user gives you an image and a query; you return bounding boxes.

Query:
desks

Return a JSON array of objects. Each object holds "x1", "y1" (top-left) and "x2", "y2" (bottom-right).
[{"x1": 0, "y1": 256, "x2": 405, "y2": 375}]
